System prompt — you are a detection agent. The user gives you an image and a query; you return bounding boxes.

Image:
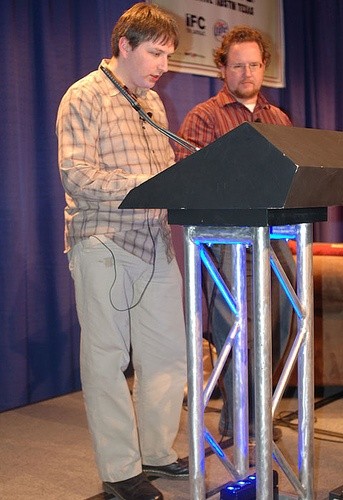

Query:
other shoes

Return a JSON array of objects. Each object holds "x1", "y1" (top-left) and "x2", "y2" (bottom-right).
[
  {"x1": 248, "y1": 427, "x2": 282, "y2": 443},
  {"x1": 219, "y1": 426, "x2": 234, "y2": 436}
]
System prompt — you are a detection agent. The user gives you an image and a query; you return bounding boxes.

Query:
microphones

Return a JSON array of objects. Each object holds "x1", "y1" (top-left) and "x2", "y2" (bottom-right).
[{"x1": 100, "y1": 65, "x2": 199, "y2": 153}]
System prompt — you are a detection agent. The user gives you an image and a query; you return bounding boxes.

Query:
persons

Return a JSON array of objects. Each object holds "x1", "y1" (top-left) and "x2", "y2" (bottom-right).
[
  {"x1": 55, "y1": 4, "x2": 187, "y2": 500},
  {"x1": 170, "y1": 28, "x2": 297, "y2": 442}
]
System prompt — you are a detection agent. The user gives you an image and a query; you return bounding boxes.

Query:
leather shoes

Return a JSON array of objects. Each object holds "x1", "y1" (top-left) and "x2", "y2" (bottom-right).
[
  {"x1": 142, "y1": 456, "x2": 197, "y2": 480},
  {"x1": 103, "y1": 471, "x2": 163, "y2": 500}
]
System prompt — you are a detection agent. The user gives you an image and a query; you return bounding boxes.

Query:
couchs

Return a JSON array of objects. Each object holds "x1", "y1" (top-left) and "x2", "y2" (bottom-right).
[{"x1": 241, "y1": 241, "x2": 343, "y2": 389}]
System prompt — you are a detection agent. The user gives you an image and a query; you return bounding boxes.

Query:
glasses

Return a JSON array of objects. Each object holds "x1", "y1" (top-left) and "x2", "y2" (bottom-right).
[{"x1": 225, "y1": 62, "x2": 265, "y2": 70}]
